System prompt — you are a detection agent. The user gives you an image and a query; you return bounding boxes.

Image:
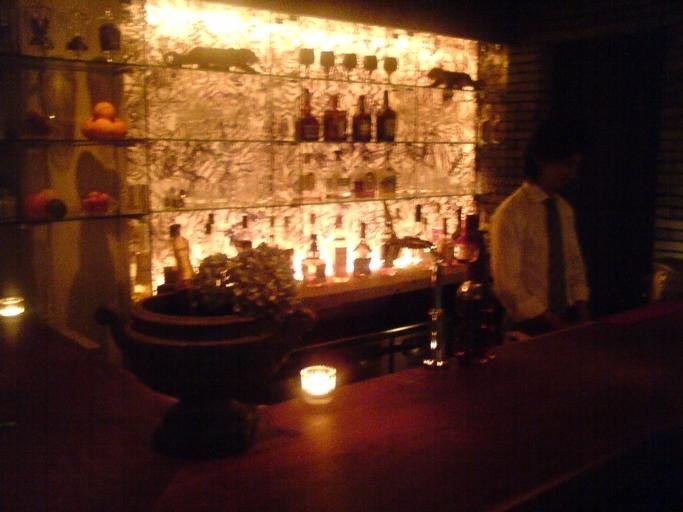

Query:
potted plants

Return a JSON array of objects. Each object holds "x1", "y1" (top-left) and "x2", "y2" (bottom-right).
[{"x1": 90, "y1": 241, "x2": 317, "y2": 459}]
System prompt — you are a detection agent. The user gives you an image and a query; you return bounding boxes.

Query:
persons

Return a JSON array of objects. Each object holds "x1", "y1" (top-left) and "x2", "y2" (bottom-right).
[{"x1": 488, "y1": 123, "x2": 592, "y2": 336}]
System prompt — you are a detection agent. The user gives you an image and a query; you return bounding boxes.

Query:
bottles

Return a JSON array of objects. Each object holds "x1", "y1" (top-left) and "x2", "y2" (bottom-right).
[
  {"x1": 198, "y1": 204, "x2": 484, "y2": 286},
  {"x1": 98, "y1": 10, "x2": 121, "y2": 60},
  {"x1": 172, "y1": 231, "x2": 195, "y2": 279},
  {"x1": 296, "y1": 89, "x2": 397, "y2": 200}
]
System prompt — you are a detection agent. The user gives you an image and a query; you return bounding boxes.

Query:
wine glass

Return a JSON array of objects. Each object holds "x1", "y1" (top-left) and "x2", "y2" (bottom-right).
[{"x1": 299, "y1": 47, "x2": 397, "y2": 84}]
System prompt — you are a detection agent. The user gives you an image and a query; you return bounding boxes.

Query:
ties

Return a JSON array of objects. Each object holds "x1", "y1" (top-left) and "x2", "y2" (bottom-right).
[{"x1": 543, "y1": 197, "x2": 567, "y2": 312}]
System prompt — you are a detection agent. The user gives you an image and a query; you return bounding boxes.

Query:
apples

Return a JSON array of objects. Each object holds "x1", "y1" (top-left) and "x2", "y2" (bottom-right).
[{"x1": 80, "y1": 102, "x2": 130, "y2": 140}]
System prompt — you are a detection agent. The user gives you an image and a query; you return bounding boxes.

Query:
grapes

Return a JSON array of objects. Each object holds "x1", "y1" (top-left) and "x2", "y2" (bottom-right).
[{"x1": 81, "y1": 190, "x2": 112, "y2": 215}]
